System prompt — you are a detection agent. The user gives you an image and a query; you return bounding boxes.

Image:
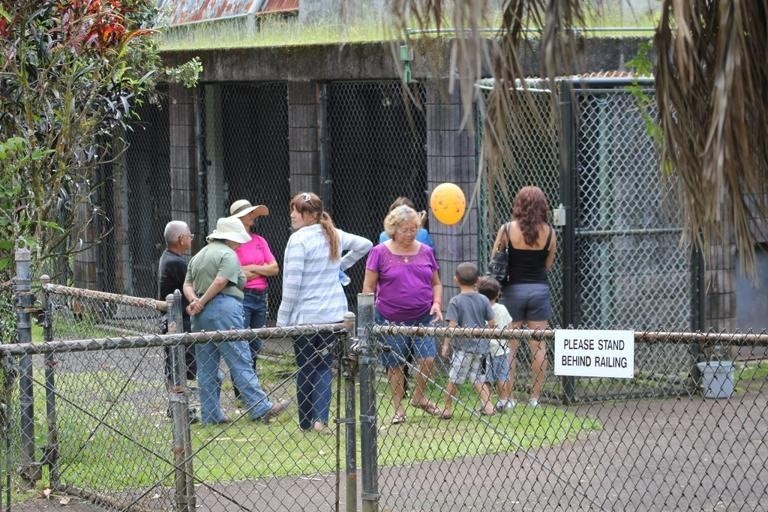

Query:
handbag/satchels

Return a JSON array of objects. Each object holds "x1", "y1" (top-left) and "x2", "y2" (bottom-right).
[{"x1": 487, "y1": 250, "x2": 509, "y2": 283}]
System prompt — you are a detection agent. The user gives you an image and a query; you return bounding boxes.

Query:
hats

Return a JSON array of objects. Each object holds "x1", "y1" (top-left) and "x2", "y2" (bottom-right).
[
  {"x1": 208, "y1": 218, "x2": 251, "y2": 244},
  {"x1": 228, "y1": 199, "x2": 268, "y2": 219}
]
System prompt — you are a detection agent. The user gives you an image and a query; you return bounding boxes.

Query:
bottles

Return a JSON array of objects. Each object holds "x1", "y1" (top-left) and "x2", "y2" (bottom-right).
[{"x1": 339, "y1": 270, "x2": 351, "y2": 287}]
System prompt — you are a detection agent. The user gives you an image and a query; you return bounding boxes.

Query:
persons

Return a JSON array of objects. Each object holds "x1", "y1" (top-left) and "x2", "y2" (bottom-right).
[
  {"x1": 379, "y1": 197, "x2": 436, "y2": 398},
  {"x1": 158, "y1": 220, "x2": 198, "y2": 423},
  {"x1": 276, "y1": 192, "x2": 373, "y2": 434},
  {"x1": 440, "y1": 262, "x2": 497, "y2": 418},
  {"x1": 230, "y1": 199, "x2": 280, "y2": 416},
  {"x1": 490, "y1": 186, "x2": 558, "y2": 409},
  {"x1": 181, "y1": 217, "x2": 291, "y2": 425},
  {"x1": 475, "y1": 277, "x2": 513, "y2": 411},
  {"x1": 362, "y1": 205, "x2": 445, "y2": 423}
]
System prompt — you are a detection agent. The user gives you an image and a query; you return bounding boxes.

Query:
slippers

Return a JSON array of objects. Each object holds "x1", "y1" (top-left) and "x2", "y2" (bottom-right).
[{"x1": 392, "y1": 399, "x2": 540, "y2": 424}]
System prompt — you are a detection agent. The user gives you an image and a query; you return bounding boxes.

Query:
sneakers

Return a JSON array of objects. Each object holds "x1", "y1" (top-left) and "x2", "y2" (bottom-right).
[{"x1": 166, "y1": 398, "x2": 330, "y2": 435}]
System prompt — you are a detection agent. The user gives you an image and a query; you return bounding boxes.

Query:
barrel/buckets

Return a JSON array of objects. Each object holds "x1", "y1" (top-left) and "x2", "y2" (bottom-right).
[{"x1": 699, "y1": 363, "x2": 737, "y2": 399}]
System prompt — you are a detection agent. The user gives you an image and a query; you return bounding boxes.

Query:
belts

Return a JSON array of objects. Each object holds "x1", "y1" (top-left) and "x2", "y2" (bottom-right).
[{"x1": 245, "y1": 289, "x2": 267, "y2": 295}]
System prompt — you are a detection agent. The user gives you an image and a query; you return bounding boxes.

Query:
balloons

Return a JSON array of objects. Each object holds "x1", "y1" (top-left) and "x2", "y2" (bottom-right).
[{"x1": 430, "y1": 183, "x2": 466, "y2": 226}]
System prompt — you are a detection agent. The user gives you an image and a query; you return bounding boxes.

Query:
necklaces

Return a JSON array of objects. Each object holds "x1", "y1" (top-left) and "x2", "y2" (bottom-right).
[{"x1": 393, "y1": 242, "x2": 419, "y2": 263}]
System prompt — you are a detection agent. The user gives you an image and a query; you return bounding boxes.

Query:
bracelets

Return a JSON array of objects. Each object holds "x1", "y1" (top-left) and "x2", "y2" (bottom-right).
[
  {"x1": 189, "y1": 297, "x2": 196, "y2": 303},
  {"x1": 435, "y1": 300, "x2": 442, "y2": 305}
]
